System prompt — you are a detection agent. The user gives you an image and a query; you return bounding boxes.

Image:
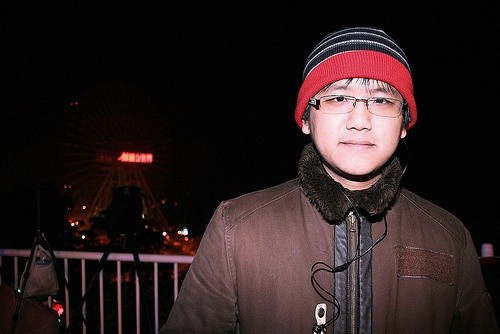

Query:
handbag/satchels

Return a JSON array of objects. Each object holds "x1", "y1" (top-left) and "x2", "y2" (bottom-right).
[{"x1": 18, "y1": 243, "x2": 60, "y2": 299}]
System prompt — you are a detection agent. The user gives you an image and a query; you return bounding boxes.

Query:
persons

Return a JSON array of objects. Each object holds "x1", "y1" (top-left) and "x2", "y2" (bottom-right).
[{"x1": 156, "y1": 24, "x2": 500, "y2": 334}]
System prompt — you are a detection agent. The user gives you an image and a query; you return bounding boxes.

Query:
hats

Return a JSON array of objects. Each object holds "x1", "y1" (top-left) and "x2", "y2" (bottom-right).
[{"x1": 294, "y1": 27, "x2": 417, "y2": 129}]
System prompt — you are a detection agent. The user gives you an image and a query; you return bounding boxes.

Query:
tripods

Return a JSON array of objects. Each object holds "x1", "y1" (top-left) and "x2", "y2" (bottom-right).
[
  {"x1": 67, "y1": 231, "x2": 159, "y2": 334},
  {"x1": 11, "y1": 191, "x2": 88, "y2": 334}
]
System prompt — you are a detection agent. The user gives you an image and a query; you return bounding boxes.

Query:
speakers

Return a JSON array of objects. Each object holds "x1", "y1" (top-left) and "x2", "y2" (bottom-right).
[{"x1": 112, "y1": 183, "x2": 146, "y2": 231}]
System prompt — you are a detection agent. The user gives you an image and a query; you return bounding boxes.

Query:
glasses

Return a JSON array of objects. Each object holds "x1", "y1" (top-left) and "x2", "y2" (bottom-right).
[{"x1": 308, "y1": 95, "x2": 408, "y2": 118}]
